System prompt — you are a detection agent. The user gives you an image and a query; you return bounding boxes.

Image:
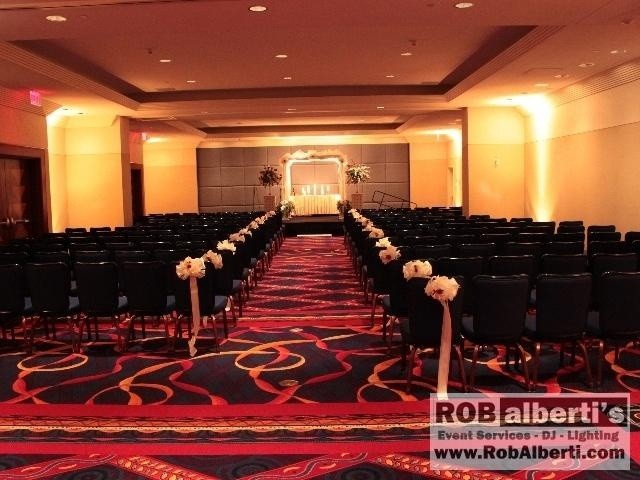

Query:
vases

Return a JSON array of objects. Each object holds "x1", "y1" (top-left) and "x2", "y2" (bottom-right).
[
  {"x1": 355, "y1": 182, "x2": 359, "y2": 192},
  {"x1": 267, "y1": 184, "x2": 272, "y2": 195}
]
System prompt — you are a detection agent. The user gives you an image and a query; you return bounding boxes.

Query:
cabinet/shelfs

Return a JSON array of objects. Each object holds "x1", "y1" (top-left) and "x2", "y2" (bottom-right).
[
  {"x1": 352, "y1": 193, "x2": 364, "y2": 208},
  {"x1": 263, "y1": 195, "x2": 277, "y2": 210}
]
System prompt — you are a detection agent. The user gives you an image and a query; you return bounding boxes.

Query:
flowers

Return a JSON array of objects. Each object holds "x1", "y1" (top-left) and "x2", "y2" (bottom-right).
[
  {"x1": 345, "y1": 161, "x2": 370, "y2": 184},
  {"x1": 280, "y1": 200, "x2": 296, "y2": 215},
  {"x1": 257, "y1": 165, "x2": 281, "y2": 187},
  {"x1": 337, "y1": 200, "x2": 350, "y2": 212}
]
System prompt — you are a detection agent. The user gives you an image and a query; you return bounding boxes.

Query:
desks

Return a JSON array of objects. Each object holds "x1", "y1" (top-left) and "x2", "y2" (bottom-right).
[{"x1": 290, "y1": 194, "x2": 340, "y2": 215}]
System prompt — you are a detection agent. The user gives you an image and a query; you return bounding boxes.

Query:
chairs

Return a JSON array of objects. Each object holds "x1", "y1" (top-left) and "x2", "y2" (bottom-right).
[
  {"x1": 341, "y1": 206, "x2": 639, "y2": 395},
  {"x1": 0, "y1": 213, "x2": 285, "y2": 355}
]
